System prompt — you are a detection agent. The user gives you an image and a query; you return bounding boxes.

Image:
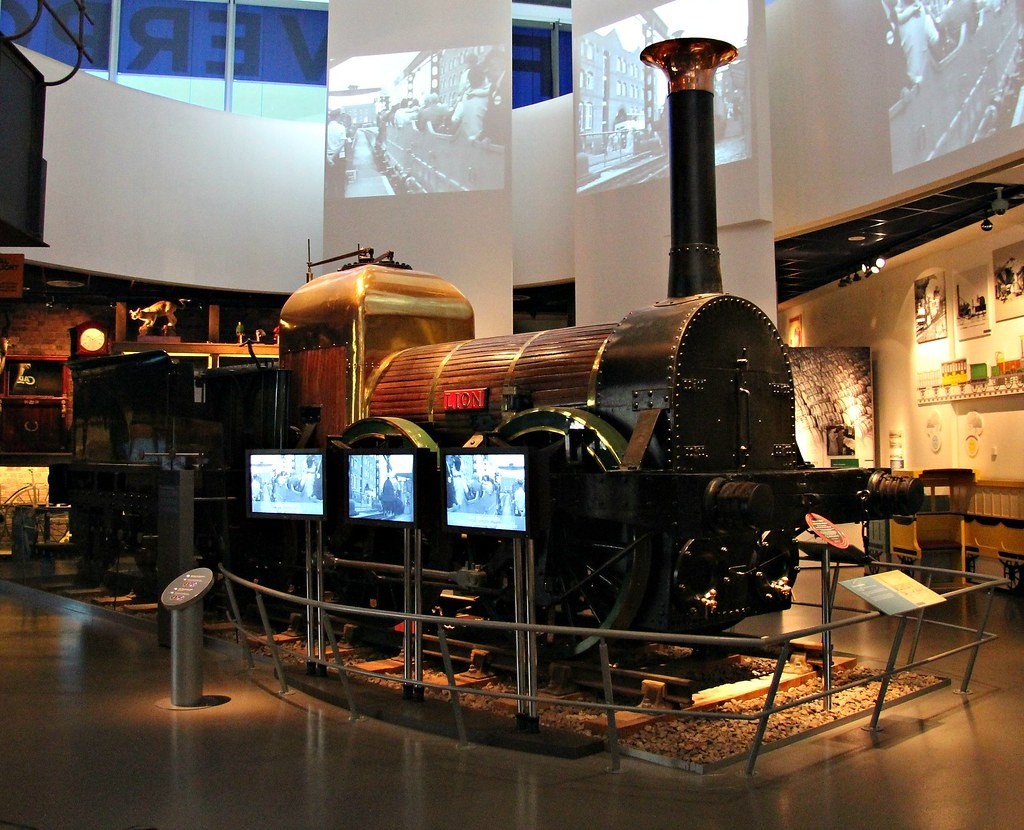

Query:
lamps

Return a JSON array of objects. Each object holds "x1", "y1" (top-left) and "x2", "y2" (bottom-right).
[
  {"x1": 837, "y1": 278, "x2": 847, "y2": 287},
  {"x1": 981, "y1": 218, "x2": 993, "y2": 231},
  {"x1": 845, "y1": 275, "x2": 853, "y2": 284},
  {"x1": 853, "y1": 272, "x2": 862, "y2": 282}
]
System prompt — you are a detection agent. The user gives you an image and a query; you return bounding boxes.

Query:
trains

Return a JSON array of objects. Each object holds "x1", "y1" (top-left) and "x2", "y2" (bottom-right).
[{"x1": 48, "y1": 38, "x2": 925, "y2": 665}]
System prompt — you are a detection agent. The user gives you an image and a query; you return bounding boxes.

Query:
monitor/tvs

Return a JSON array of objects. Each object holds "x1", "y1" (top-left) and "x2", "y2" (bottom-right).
[
  {"x1": 439, "y1": 446, "x2": 531, "y2": 540},
  {"x1": 244, "y1": 449, "x2": 329, "y2": 521},
  {"x1": 343, "y1": 448, "x2": 426, "y2": 528}
]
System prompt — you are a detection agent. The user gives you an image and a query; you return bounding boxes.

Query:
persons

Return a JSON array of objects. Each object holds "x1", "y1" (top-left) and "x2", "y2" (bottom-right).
[
  {"x1": 327, "y1": 108, "x2": 354, "y2": 193},
  {"x1": 377, "y1": 48, "x2": 508, "y2": 146},
  {"x1": 878, "y1": 0, "x2": 1015, "y2": 108},
  {"x1": 446, "y1": 455, "x2": 525, "y2": 516},
  {"x1": 383, "y1": 476, "x2": 395, "y2": 494},
  {"x1": 251, "y1": 455, "x2": 323, "y2": 502}
]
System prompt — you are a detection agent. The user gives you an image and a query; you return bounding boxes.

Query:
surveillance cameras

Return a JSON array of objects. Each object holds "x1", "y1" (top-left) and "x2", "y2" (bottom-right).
[{"x1": 991, "y1": 199, "x2": 1009, "y2": 215}]
